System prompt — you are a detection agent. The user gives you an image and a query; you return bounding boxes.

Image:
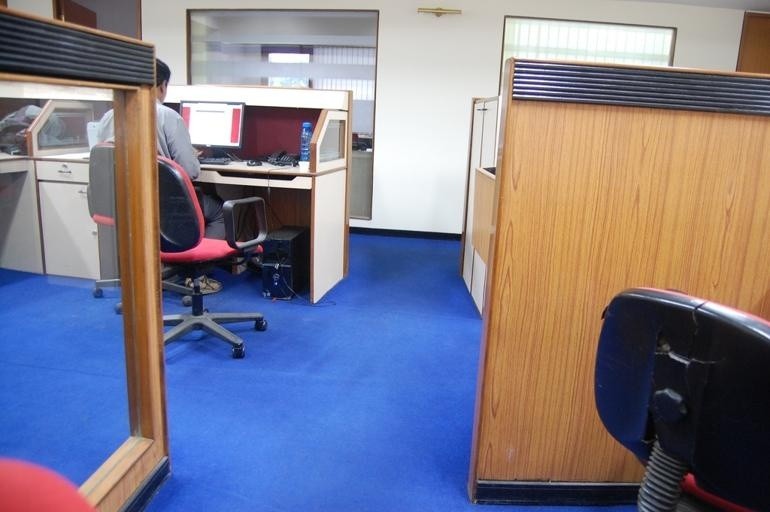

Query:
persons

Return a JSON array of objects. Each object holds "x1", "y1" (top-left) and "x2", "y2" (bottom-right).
[{"x1": 97, "y1": 58, "x2": 228, "y2": 296}]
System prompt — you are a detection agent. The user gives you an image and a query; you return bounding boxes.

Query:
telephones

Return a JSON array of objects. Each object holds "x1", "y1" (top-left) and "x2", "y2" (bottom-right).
[{"x1": 275, "y1": 150, "x2": 300, "y2": 165}]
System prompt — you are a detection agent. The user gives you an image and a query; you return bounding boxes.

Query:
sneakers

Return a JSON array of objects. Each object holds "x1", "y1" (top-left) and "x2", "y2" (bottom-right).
[{"x1": 184, "y1": 278, "x2": 225, "y2": 295}]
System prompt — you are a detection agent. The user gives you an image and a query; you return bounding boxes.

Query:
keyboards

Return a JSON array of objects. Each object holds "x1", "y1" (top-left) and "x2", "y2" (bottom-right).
[{"x1": 199, "y1": 157, "x2": 229, "y2": 164}]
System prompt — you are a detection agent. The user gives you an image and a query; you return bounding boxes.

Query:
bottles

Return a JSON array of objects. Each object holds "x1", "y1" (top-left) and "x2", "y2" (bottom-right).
[{"x1": 300, "y1": 121, "x2": 313, "y2": 161}]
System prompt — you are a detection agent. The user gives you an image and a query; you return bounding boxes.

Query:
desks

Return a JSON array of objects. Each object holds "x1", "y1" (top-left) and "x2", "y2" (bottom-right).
[{"x1": 35, "y1": 79, "x2": 359, "y2": 303}]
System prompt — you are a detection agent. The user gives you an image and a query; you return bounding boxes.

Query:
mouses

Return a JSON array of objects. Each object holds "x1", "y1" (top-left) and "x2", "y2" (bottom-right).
[{"x1": 247, "y1": 159, "x2": 262, "y2": 166}]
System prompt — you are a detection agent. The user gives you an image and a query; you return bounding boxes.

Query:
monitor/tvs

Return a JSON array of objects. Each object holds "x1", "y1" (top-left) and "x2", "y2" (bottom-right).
[{"x1": 179, "y1": 100, "x2": 246, "y2": 157}]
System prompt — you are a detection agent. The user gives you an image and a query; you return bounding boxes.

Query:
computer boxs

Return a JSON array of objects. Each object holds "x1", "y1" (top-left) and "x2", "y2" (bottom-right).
[{"x1": 261, "y1": 226, "x2": 310, "y2": 300}]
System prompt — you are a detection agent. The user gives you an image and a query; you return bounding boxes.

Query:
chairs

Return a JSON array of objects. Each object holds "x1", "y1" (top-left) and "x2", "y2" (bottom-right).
[
  {"x1": 81, "y1": 138, "x2": 199, "y2": 313},
  {"x1": 154, "y1": 155, "x2": 275, "y2": 358},
  {"x1": 590, "y1": 279, "x2": 770, "y2": 512}
]
